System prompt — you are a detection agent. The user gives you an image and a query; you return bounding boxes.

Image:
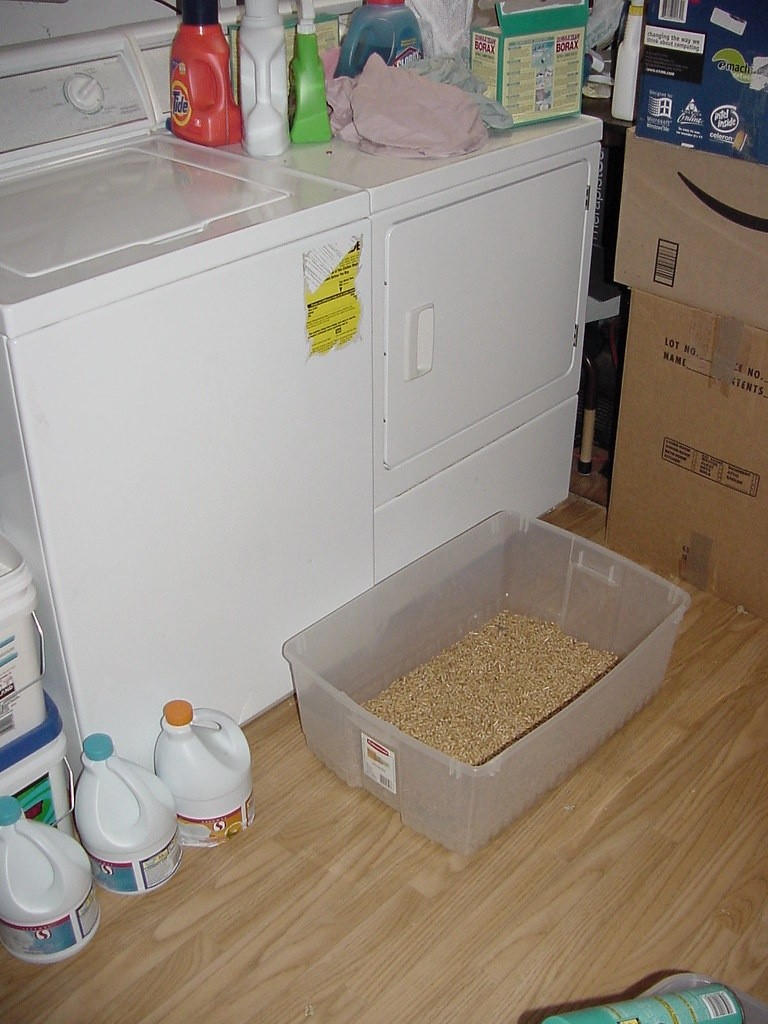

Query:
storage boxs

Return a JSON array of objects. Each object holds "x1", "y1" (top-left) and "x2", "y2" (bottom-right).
[
  {"x1": 469, "y1": 0, "x2": 588, "y2": 128},
  {"x1": 635, "y1": 973, "x2": 768, "y2": 1024},
  {"x1": 282, "y1": 510, "x2": 691, "y2": 851},
  {"x1": 227, "y1": 13, "x2": 340, "y2": 105},
  {"x1": 606, "y1": 0, "x2": 768, "y2": 622}
]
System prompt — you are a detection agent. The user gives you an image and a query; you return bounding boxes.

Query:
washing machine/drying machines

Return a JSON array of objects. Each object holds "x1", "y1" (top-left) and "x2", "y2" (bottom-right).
[{"x1": 0, "y1": 26, "x2": 378, "y2": 793}]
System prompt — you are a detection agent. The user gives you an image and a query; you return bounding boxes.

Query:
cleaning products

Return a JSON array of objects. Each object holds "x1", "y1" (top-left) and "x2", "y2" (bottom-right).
[
  {"x1": 610, "y1": 0, "x2": 646, "y2": 123},
  {"x1": 288, "y1": 0, "x2": 332, "y2": 145},
  {"x1": 238, "y1": 0, "x2": 291, "y2": 160},
  {"x1": 169, "y1": 1, "x2": 242, "y2": 148},
  {"x1": 331, "y1": 0, "x2": 425, "y2": 79}
]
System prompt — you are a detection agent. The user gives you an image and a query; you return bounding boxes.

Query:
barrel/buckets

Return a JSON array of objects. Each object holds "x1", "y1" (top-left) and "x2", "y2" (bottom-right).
[
  {"x1": 0, "y1": 531, "x2": 47, "y2": 748},
  {"x1": 0, "y1": 688, "x2": 76, "y2": 839}
]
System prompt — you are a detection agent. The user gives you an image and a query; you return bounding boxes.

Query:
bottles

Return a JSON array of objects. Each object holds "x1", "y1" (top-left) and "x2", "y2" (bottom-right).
[
  {"x1": 170, "y1": 0, "x2": 290, "y2": 156},
  {"x1": 153, "y1": 700, "x2": 253, "y2": 846},
  {"x1": 611, "y1": 0, "x2": 644, "y2": 122},
  {"x1": 74, "y1": 733, "x2": 183, "y2": 894},
  {"x1": 1, "y1": 796, "x2": 100, "y2": 964},
  {"x1": 334, "y1": 0, "x2": 424, "y2": 78}
]
